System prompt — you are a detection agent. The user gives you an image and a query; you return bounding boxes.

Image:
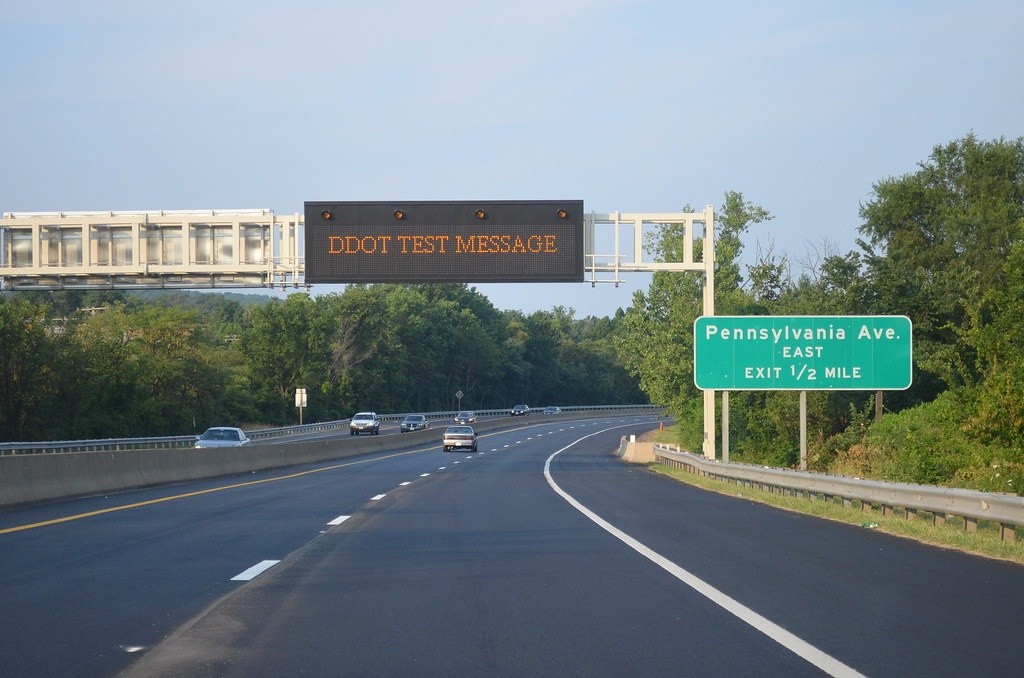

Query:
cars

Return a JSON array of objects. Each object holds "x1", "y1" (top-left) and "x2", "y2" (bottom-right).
[
  {"x1": 541, "y1": 406, "x2": 561, "y2": 415},
  {"x1": 193, "y1": 427, "x2": 250, "y2": 447},
  {"x1": 443, "y1": 427, "x2": 479, "y2": 451},
  {"x1": 400, "y1": 416, "x2": 429, "y2": 433},
  {"x1": 455, "y1": 412, "x2": 477, "y2": 424},
  {"x1": 511, "y1": 405, "x2": 531, "y2": 417}
]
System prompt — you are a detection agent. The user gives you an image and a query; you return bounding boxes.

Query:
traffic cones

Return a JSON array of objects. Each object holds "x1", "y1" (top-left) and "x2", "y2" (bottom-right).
[{"x1": 659, "y1": 422, "x2": 663, "y2": 431}]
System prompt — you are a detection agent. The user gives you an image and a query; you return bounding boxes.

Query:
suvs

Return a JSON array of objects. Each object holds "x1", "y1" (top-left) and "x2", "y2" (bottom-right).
[{"x1": 349, "y1": 412, "x2": 380, "y2": 435}]
